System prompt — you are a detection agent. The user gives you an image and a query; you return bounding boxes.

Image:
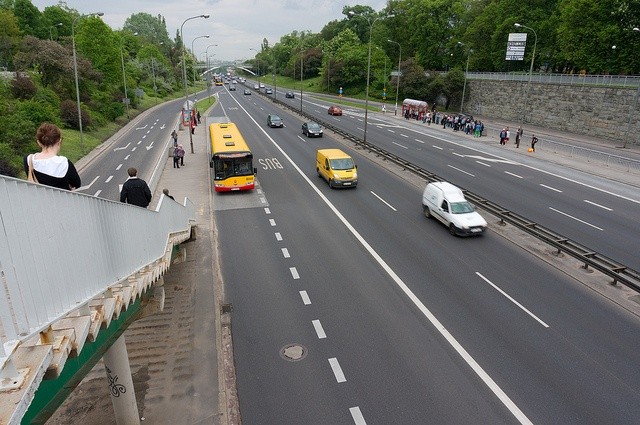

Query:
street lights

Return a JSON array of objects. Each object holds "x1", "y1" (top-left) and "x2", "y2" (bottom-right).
[
  {"x1": 348, "y1": 11, "x2": 396, "y2": 148},
  {"x1": 55, "y1": 9, "x2": 104, "y2": 158},
  {"x1": 616, "y1": 27, "x2": 640, "y2": 149},
  {"x1": 206, "y1": 44, "x2": 219, "y2": 102},
  {"x1": 191, "y1": 35, "x2": 209, "y2": 124},
  {"x1": 457, "y1": 41, "x2": 472, "y2": 115},
  {"x1": 118, "y1": 29, "x2": 138, "y2": 118},
  {"x1": 513, "y1": 23, "x2": 537, "y2": 149},
  {"x1": 179, "y1": 14, "x2": 210, "y2": 152},
  {"x1": 386, "y1": 38, "x2": 403, "y2": 115}
]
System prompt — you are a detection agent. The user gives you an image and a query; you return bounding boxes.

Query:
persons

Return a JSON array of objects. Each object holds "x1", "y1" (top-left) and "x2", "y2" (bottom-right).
[
  {"x1": 119, "y1": 168, "x2": 152, "y2": 209},
  {"x1": 171, "y1": 110, "x2": 201, "y2": 146},
  {"x1": 172, "y1": 144, "x2": 185, "y2": 168},
  {"x1": 404, "y1": 102, "x2": 538, "y2": 152},
  {"x1": 24, "y1": 124, "x2": 81, "y2": 192},
  {"x1": 163, "y1": 188, "x2": 176, "y2": 200}
]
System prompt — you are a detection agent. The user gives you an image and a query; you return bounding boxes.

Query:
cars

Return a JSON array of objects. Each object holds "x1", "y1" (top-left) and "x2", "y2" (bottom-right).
[
  {"x1": 244, "y1": 88, "x2": 252, "y2": 95},
  {"x1": 265, "y1": 88, "x2": 273, "y2": 94},
  {"x1": 267, "y1": 115, "x2": 284, "y2": 129},
  {"x1": 328, "y1": 106, "x2": 342, "y2": 116},
  {"x1": 229, "y1": 85, "x2": 236, "y2": 91},
  {"x1": 302, "y1": 121, "x2": 323, "y2": 138},
  {"x1": 286, "y1": 91, "x2": 294, "y2": 99}
]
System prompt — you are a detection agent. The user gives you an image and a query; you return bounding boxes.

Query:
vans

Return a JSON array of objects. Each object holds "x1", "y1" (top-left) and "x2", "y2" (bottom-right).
[{"x1": 316, "y1": 148, "x2": 359, "y2": 189}]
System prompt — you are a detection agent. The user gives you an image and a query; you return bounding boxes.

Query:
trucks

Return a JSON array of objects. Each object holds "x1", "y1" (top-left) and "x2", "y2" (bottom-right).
[{"x1": 421, "y1": 180, "x2": 488, "y2": 239}]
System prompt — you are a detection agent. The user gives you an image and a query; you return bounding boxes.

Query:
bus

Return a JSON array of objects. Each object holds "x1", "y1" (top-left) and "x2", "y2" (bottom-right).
[
  {"x1": 215, "y1": 77, "x2": 223, "y2": 86},
  {"x1": 209, "y1": 122, "x2": 257, "y2": 194}
]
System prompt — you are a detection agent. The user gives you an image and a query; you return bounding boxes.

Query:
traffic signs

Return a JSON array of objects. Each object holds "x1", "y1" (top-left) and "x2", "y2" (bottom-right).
[{"x1": 504, "y1": 32, "x2": 528, "y2": 61}]
